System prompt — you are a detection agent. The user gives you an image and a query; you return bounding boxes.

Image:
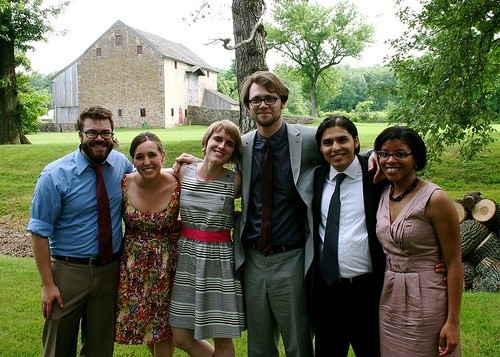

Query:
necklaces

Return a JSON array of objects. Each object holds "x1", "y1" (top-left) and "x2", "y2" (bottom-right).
[{"x1": 389, "y1": 178, "x2": 419, "y2": 202}]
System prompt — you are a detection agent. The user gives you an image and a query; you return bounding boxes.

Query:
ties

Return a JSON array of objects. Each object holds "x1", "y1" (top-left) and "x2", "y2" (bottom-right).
[
  {"x1": 321, "y1": 173, "x2": 348, "y2": 286},
  {"x1": 90, "y1": 163, "x2": 114, "y2": 264},
  {"x1": 259, "y1": 139, "x2": 275, "y2": 257}
]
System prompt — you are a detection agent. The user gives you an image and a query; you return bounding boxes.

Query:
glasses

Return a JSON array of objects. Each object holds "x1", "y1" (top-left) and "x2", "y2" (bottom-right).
[
  {"x1": 248, "y1": 94, "x2": 280, "y2": 106},
  {"x1": 81, "y1": 129, "x2": 114, "y2": 139},
  {"x1": 378, "y1": 150, "x2": 413, "y2": 159}
]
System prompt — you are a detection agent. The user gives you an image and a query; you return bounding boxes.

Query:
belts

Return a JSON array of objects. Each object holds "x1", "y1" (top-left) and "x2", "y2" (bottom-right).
[
  {"x1": 245, "y1": 240, "x2": 306, "y2": 253},
  {"x1": 52, "y1": 252, "x2": 120, "y2": 265},
  {"x1": 339, "y1": 272, "x2": 372, "y2": 284}
]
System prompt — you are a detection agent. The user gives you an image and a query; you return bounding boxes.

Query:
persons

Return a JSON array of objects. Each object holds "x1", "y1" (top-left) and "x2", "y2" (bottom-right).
[
  {"x1": 303, "y1": 116, "x2": 446, "y2": 357},
  {"x1": 159, "y1": 120, "x2": 242, "y2": 357},
  {"x1": 171, "y1": 72, "x2": 389, "y2": 357},
  {"x1": 374, "y1": 126, "x2": 465, "y2": 357},
  {"x1": 113, "y1": 133, "x2": 241, "y2": 357},
  {"x1": 26, "y1": 104, "x2": 179, "y2": 357}
]
